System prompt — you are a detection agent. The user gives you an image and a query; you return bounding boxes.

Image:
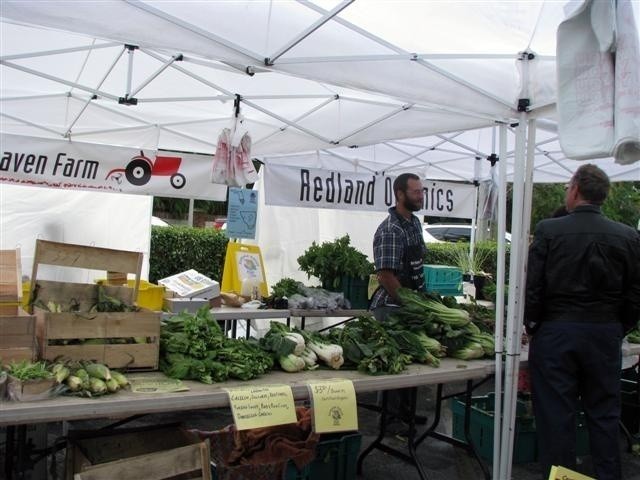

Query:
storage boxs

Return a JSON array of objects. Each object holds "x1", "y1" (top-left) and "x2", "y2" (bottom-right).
[{"x1": 0, "y1": 303, "x2": 162, "y2": 373}]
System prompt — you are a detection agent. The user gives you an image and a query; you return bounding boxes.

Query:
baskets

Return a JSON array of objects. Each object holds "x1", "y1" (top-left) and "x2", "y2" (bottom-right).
[
  {"x1": 322, "y1": 274, "x2": 368, "y2": 309},
  {"x1": 422, "y1": 264, "x2": 463, "y2": 296}
]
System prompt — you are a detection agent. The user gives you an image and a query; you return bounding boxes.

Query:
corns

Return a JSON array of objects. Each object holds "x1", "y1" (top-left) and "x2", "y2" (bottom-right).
[{"x1": 51, "y1": 362, "x2": 130, "y2": 393}]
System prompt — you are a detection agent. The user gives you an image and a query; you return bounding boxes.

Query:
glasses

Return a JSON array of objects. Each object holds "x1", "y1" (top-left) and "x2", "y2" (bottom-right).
[
  {"x1": 561, "y1": 184, "x2": 571, "y2": 190},
  {"x1": 406, "y1": 190, "x2": 424, "y2": 193}
]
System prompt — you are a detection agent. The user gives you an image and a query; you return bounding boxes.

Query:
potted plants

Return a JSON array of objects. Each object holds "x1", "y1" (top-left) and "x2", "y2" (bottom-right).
[{"x1": 441, "y1": 232, "x2": 499, "y2": 298}]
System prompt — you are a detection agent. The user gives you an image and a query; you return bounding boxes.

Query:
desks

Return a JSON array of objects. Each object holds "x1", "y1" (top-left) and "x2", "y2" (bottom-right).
[
  {"x1": 1, "y1": 331, "x2": 640, "y2": 479},
  {"x1": 156, "y1": 295, "x2": 497, "y2": 340}
]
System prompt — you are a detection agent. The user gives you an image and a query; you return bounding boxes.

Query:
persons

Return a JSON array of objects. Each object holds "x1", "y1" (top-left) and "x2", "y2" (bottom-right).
[
  {"x1": 523, "y1": 164, "x2": 640, "y2": 480},
  {"x1": 369, "y1": 172, "x2": 428, "y2": 437}
]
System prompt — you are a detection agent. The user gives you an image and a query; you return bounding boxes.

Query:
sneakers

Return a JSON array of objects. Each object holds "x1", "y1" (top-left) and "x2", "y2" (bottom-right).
[{"x1": 377, "y1": 410, "x2": 428, "y2": 436}]
